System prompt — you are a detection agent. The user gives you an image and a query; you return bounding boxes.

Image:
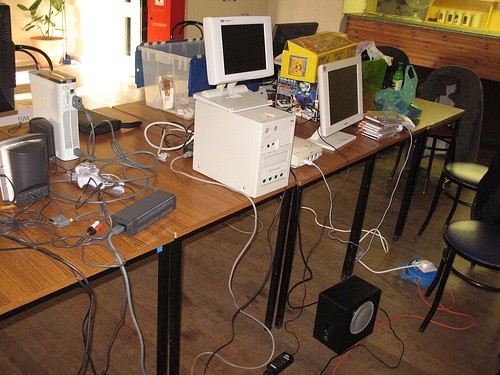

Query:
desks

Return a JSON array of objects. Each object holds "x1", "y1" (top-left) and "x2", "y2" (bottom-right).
[
  {"x1": 0, "y1": 92, "x2": 465, "y2": 375},
  {"x1": 346, "y1": 13, "x2": 500, "y2": 82}
]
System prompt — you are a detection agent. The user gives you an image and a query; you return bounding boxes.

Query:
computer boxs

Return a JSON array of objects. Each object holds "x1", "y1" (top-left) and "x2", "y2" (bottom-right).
[
  {"x1": 29, "y1": 69, "x2": 81, "y2": 162},
  {"x1": 192, "y1": 100, "x2": 297, "y2": 199}
]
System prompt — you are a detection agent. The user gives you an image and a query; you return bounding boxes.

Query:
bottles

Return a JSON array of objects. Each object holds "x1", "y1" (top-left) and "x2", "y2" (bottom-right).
[{"x1": 391, "y1": 62, "x2": 404, "y2": 90}]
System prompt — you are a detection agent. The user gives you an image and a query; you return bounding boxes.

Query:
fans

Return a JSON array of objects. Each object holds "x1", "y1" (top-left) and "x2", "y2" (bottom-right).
[{"x1": 403, "y1": 0, "x2": 435, "y2": 22}]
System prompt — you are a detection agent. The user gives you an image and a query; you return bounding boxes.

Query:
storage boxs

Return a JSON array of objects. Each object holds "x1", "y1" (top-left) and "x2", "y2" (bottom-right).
[
  {"x1": 135, "y1": 40, "x2": 262, "y2": 120},
  {"x1": 280, "y1": 31, "x2": 358, "y2": 83},
  {"x1": 361, "y1": 59, "x2": 388, "y2": 92}
]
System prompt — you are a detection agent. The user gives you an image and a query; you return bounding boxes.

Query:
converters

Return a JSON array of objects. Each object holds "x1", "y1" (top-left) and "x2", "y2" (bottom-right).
[
  {"x1": 110, "y1": 189, "x2": 177, "y2": 237},
  {"x1": 267, "y1": 352, "x2": 294, "y2": 375}
]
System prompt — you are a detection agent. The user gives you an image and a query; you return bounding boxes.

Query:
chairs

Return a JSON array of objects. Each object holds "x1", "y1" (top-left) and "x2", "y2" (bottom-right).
[{"x1": 361, "y1": 46, "x2": 500, "y2": 333}]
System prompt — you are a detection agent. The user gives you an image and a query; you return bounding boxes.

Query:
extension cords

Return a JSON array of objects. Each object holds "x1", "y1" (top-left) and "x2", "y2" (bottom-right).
[{"x1": 397, "y1": 257, "x2": 439, "y2": 286}]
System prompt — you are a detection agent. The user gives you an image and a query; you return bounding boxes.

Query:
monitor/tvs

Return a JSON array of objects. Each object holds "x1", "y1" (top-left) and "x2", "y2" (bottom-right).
[
  {"x1": 272, "y1": 22, "x2": 320, "y2": 58},
  {"x1": 193, "y1": 16, "x2": 275, "y2": 113},
  {"x1": 0, "y1": 3, "x2": 16, "y2": 113},
  {"x1": 307, "y1": 55, "x2": 364, "y2": 152}
]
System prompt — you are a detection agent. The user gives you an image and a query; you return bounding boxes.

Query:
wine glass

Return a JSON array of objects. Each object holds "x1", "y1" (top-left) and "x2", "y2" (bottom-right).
[{"x1": 405, "y1": 0, "x2": 435, "y2": 22}]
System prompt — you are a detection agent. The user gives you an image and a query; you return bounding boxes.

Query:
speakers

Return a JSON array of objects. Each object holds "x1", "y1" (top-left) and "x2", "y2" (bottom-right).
[
  {"x1": 0, "y1": 117, "x2": 57, "y2": 206},
  {"x1": 313, "y1": 275, "x2": 382, "y2": 355}
]
